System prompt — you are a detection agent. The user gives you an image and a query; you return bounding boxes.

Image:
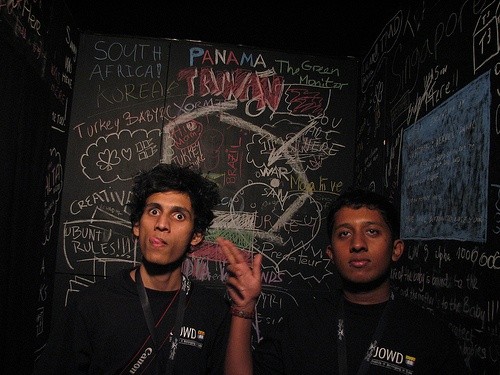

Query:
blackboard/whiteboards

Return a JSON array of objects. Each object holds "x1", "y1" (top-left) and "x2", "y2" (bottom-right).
[
  {"x1": 0, "y1": 0, "x2": 51, "y2": 77},
  {"x1": 54, "y1": 31, "x2": 359, "y2": 356},
  {"x1": 27, "y1": 0, "x2": 80, "y2": 370},
  {"x1": 359, "y1": 2, "x2": 500, "y2": 374}
]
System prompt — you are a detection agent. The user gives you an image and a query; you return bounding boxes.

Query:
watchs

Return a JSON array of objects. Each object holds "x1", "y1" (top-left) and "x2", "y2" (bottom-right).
[{"x1": 232, "y1": 308, "x2": 257, "y2": 319}]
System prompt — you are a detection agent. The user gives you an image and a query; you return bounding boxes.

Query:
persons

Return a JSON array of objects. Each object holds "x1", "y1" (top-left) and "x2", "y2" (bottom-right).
[
  {"x1": 217, "y1": 198, "x2": 462, "y2": 375},
  {"x1": 35, "y1": 162, "x2": 243, "y2": 374}
]
